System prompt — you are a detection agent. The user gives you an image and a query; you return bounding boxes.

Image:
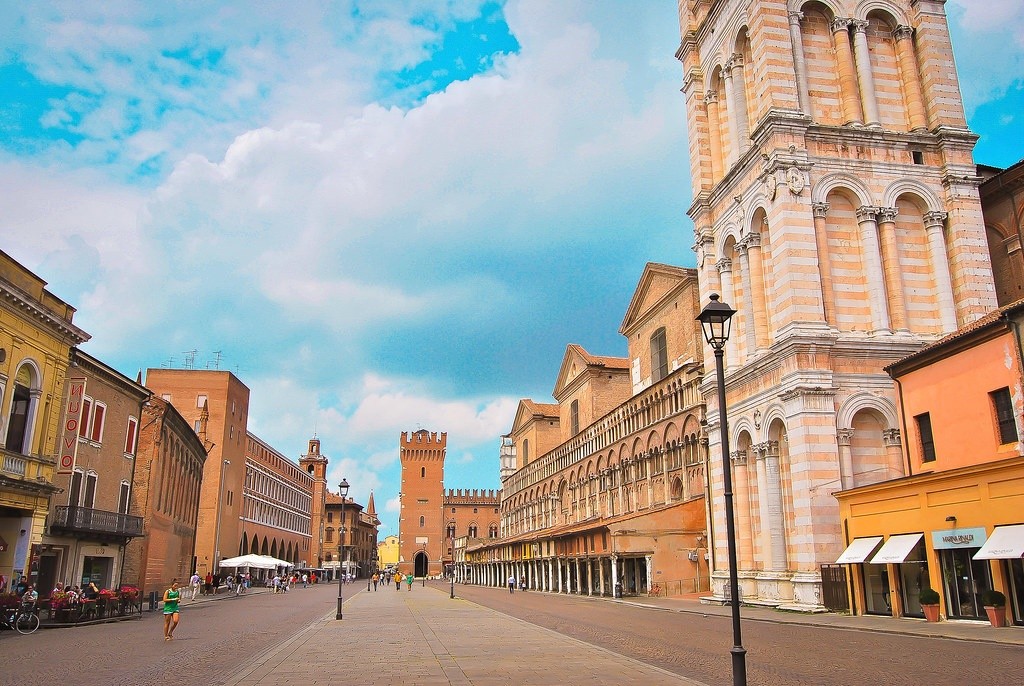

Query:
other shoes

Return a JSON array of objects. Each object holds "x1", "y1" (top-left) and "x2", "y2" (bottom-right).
[
  {"x1": 164, "y1": 637, "x2": 170, "y2": 640},
  {"x1": 168, "y1": 634, "x2": 173, "y2": 640},
  {"x1": 192, "y1": 600, "x2": 195, "y2": 601}
]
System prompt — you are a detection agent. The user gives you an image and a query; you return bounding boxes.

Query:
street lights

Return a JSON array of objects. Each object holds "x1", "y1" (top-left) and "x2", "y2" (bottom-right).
[
  {"x1": 423, "y1": 541, "x2": 426, "y2": 587},
  {"x1": 449, "y1": 518, "x2": 456, "y2": 600},
  {"x1": 336, "y1": 478, "x2": 351, "y2": 620},
  {"x1": 695, "y1": 292, "x2": 748, "y2": 686},
  {"x1": 367, "y1": 533, "x2": 373, "y2": 592}
]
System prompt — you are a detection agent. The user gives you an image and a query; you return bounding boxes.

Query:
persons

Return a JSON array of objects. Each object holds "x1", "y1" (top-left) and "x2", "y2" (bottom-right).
[
  {"x1": 189, "y1": 571, "x2": 201, "y2": 601},
  {"x1": 394, "y1": 571, "x2": 402, "y2": 591},
  {"x1": 508, "y1": 575, "x2": 515, "y2": 593},
  {"x1": 271, "y1": 574, "x2": 296, "y2": 594},
  {"x1": 342, "y1": 572, "x2": 356, "y2": 585},
  {"x1": 50, "y1": 581, "x2": 99, "y2": 617},
  {"x1": 380, "y1": 573, "x2": 384, "y2": 586},
  {"x1": 915, "y1": 566, "x2": 929, "y2": 613},
  {"x1": 163, "y1": 578, "x2": 180, "y2": 641},
  {"x1": 386, "y1": 571, "x2": 390, "y2": 585},
  {"x1": 880, "y1": 565, "x2": 892, "y2": 611},
  {"x1": 406, "y1": 572, "x2": 412, "y2": 591},
  {"x1": 372, "y1": 572, "x2": 379, "y2": 591},
  {"x1": 302, "y1": 573, "x2": 308, "y2": 588},
  {"x1": 425, "y1": 573, "x2": 429, "y2": 582},
  {"x1": 311, "y1": 573, "x2": 316, "y2": 585},
  {"x1": 16, "y1": 576, "x2": 28, "y2": 597},
  {"x1": 227, "y1": 571, "x2": 250, "y2": 596},
  {"x1": 212, "y1": 571, "x2": 219, "y2": 596},
  {"x1": 520, "y1": 575, "x2": 526, "y2": 591},
  {"x1": 203, "y1": 572, "x2": 212, "y2": 596},
  {"x1": 21, "y1": 584, "x2": 38, "y2": 612}
]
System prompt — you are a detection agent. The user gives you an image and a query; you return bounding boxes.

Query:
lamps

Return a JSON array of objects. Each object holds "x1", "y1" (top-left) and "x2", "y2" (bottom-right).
[
  {"x1": 944, "y1": 516, "x2": 957, "y2": 521},
  {"x1": 20, "y1": 529, "x2": 26, "y2": 535}
]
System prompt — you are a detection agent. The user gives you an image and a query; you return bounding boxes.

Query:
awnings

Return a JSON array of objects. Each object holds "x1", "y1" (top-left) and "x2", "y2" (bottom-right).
[
  {"x1": 870, "y1": 534, "x2": 923, "y2": 564},
  {"x1": 972, "y1": 525, "x2": 1024, "y2": 560},
  {"x1": 835, "y1": 537, "x2": 883, "y2": 563}
]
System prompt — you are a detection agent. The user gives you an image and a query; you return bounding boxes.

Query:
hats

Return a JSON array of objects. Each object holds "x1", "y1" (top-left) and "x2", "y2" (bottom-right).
[
  {"x1": 194, "y1": 571, "x2": 199, "y2": 574},
  {"x1": 28, "y1": 585, "x2": 34, "y2": 588}
]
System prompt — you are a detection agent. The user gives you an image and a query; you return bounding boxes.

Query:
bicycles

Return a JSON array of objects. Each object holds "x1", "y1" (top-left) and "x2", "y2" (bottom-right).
[{"x1": 1, "y1": 600, "x2": 40, "y2": 635}]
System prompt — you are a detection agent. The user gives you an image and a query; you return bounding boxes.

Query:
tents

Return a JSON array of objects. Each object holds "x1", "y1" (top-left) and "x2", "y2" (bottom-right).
[{"x1": 218, "y1": 553, "x2": 295, "y2": 587}]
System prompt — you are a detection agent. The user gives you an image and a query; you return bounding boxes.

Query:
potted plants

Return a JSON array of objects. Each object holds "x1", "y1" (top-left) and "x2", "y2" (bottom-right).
[
  {"x1": 918, "y1": 588, "x2": 939, "y2": 622},
  {"x1": 980, "y1": 590, "x2": 1006, "y2": 627}
]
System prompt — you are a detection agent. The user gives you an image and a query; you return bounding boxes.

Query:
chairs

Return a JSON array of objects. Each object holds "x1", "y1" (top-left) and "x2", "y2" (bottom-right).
[{"x1": 0, "y1": 584, "x2": 142, "y2": 624}]
